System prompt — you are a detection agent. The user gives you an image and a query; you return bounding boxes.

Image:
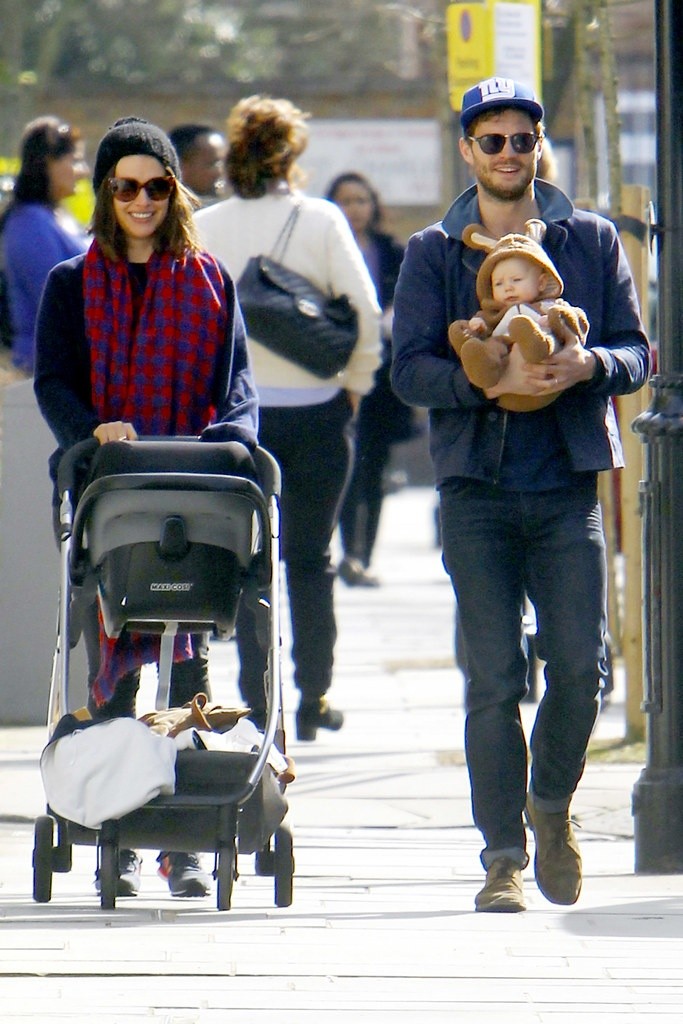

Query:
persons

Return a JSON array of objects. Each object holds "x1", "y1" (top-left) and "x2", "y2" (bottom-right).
[
  {"x1": 33, "y1": 119, "x2": 260, "y2": 897},
  {"x1": 184, "y1": 98, "x2": 382, "y2": 740},
  {"x1": 0, "y1": 115, "x2": 92, "y2": 379},
  {"x1": 390, "y1": 79, "x2": 650, "y2": 912},
  {"x1": 447, "y1": 220, "x2": 589, "y2": 409},
  {"x1": 323, "y1": 175, "x2": 425, "y2": 587},
  {"x1": 166, "y1": 123, "x2": 227, "y2": 212}
]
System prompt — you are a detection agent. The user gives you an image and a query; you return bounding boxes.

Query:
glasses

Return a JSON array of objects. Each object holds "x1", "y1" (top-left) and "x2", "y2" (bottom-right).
[
  {"x1": 109, "y1": 176, "x2": 174, "y2": 201},
  {"x1": 469, "y1": 132, "x2": 539, "y2": 155}
]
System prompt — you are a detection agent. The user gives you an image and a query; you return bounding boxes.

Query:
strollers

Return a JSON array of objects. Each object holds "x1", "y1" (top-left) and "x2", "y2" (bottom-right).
[{"x1": 33, "y1": 433, "x2": 296, "y2": 912}]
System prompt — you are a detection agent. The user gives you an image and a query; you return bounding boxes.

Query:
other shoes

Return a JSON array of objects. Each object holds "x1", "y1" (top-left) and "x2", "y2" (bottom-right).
[{"x1": 339, "y1": 560, "x2": 380, "y2": 587}]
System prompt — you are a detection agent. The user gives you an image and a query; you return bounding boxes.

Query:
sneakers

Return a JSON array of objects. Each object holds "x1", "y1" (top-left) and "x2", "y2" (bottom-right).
[
  {"x1": 475, "y1": 858, "x2": 526, "y2": 912},
  {"x1": 156, "y1": 849, "x2": 211, "y2": 897},
  {"x1": 524, "y1": 794, "x2": 583, "y2": 905},
  {"x1": 94, "y1": 848, "x2": 141, "y2": 895}
]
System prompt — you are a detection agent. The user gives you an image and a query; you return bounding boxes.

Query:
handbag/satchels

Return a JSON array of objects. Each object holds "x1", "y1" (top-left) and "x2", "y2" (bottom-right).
[{"x1": 235, "y1": 201, "x2": 359, "y2": 381}]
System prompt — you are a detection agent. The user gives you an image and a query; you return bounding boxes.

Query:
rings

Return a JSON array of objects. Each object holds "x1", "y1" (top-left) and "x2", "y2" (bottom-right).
[
  {"x1": 118, "y1": 435, "x2": 127, "y2": 441},
  {"x1": 555, "y1": 378, "x2": 558, "y2": 385}
]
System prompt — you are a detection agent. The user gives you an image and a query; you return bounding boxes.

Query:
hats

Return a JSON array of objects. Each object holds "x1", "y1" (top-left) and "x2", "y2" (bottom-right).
[
  {"x1": 92, "y1": 117, "x2": 179, "y2": 193},
  {"x1": 459, "y1": 76, "x2": 543, "y2": 137}
]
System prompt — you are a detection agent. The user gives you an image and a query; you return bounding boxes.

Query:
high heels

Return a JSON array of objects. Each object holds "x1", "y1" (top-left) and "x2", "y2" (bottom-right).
[{"x1": 295, "y1": 702, "x2": 343, "y2": 741}]
